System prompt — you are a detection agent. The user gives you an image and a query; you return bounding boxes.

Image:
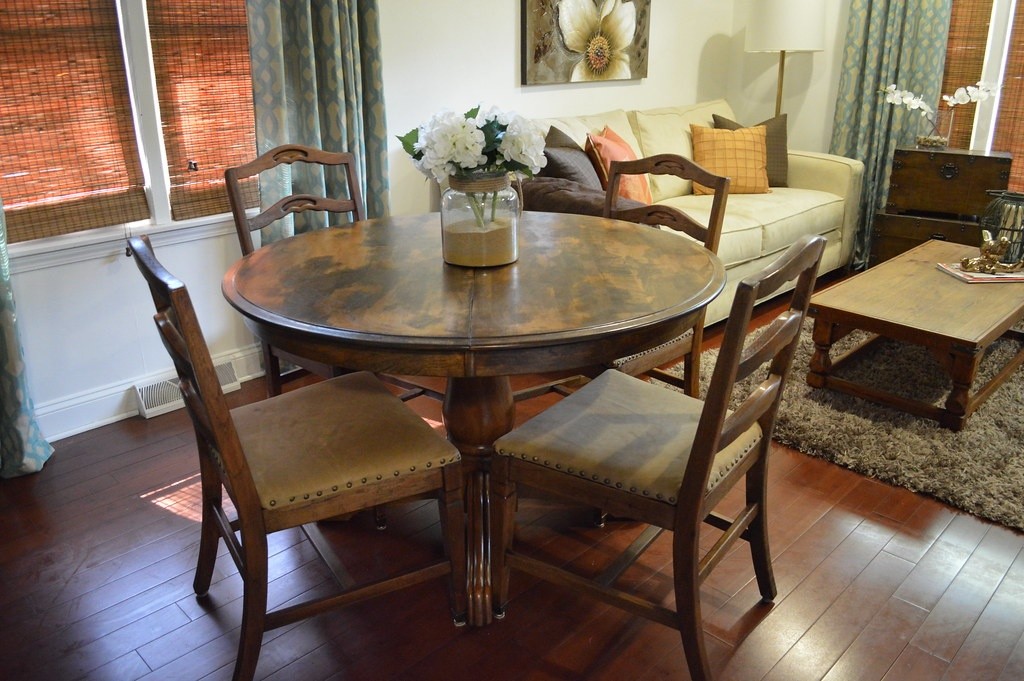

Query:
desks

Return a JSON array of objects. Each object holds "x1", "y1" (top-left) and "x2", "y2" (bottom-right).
[{"x1": 222, "y1": 208, "x2": 727, "y2": 629}]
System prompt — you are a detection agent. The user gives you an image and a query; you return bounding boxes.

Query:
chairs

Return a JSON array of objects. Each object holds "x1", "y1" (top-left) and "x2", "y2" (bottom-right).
[
  {"x1": 604, "y1": 153, "x2": 730, "y2": 400},
  {"x1": 125, "y1": 233, "x2": 467, "y2": 681},
  {"x1": 224, "y1": 143, "x2": 368, "y2": 400},
  {"x1": 490, "y1": 232, "x2": 827, "y2": 681}
]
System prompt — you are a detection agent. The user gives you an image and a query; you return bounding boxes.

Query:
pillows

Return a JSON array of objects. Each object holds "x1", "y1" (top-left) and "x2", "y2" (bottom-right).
[
  {"x1": 689, "y1": 122, "x2": 770, "y2": 194},
  {"x1": 712, "y1": 114, "x2": 789, "y2": 187},
  {"x1": 542, "y1": 125, "x2": 603, "y2": 193},
  {"x1": 585, "y1": 124, "x2": 653, "y2": 207}
]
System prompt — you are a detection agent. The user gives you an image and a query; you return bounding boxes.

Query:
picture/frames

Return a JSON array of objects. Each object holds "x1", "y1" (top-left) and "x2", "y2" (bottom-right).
[{"x1": 521, "y1": 0, "x2": 651, "y2": 85}]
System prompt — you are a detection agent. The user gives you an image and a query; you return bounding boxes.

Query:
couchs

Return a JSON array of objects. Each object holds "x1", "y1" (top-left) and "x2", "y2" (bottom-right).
[{"x1": 511, "y1": 98, "x2": 864, "y2": 328}]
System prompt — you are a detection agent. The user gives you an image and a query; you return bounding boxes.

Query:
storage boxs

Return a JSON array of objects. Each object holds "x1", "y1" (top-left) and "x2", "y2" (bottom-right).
[
  {"x1": 867, "y1": 209, "x2": 1001, "y2": 270},
  {"x1": 885, "y1": 146, "x2": 1013, "y2": 217}
]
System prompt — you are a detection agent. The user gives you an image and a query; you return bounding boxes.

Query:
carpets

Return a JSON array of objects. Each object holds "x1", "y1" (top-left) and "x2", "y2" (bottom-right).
[{"x1": 645, "y1": 307, "x2": 1024, "y2": 534}]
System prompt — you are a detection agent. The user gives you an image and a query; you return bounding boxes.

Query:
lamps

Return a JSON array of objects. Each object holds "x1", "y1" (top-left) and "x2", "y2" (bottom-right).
[{"x1": 744, "y1": 0, "x2": 825, "y2": 117}]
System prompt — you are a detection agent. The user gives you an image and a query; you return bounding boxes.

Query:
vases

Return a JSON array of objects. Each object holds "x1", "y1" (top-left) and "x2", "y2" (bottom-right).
[
  {"x1": 440, "y1": 172, "x2": 523, "y2": 268},
  {"x1": 915, "y1": 135, "x2": 949, "y2": 151}
]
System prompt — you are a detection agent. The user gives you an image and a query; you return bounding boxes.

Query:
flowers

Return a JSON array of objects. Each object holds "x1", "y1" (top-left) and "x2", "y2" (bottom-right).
[
  {"x1": 876, "y1": 82, "x2": 995, "y2": 138},
  {"x1": 395, "y1": 106, "x2": 534, "y2": 225}
]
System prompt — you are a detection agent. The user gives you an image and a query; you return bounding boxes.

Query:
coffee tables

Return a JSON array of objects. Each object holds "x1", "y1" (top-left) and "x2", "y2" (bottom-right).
[{"x1": 805, "y1": 240, "x2": 1024, "y2": 433}]
